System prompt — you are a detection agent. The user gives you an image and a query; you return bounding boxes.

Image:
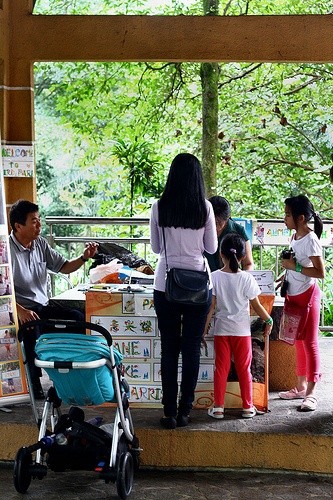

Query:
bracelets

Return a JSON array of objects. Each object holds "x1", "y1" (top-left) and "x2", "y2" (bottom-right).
[
  {"x1": 80, "y1": 256, "x2": 88, "y2": 262},
  {"x1": 295, "y1": 263, "x2": 303, "y2": 272},
  {"x1": 264, "y1": 317, "x2": 273, "y2": 326}
]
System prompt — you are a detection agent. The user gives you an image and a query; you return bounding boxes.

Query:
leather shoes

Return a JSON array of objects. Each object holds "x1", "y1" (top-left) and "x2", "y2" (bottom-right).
[{"x1": 33, "y1": 381, "x2": 45, "y2": 400}]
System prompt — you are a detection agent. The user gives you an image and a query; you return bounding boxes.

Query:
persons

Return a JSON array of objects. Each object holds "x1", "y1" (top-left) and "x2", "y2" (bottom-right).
[
  {"x1": 203, "y1": 195, "x2": 254, "y2": 273},
  {"x1": 151, "y1": 152, "x2": 219, "y2": 428},
  {"x1": 274, "y1": 194, "x2": 325, "y2": 410},
  {"x1": 202, "y1": 234, "x2": 273, "y2": 419},
  {"x1": 9, "y1": 199, "x2": 99, "y2": 400}
]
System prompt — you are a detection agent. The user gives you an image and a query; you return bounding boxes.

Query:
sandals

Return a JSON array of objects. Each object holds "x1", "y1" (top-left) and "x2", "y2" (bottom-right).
[
  {"x1": 301, "y1": 396, "x2": 317, "y2": 410},
  {"x1": 242, "y1": 406, "x2": 256, "y2": 417},
  {"x1": 279, "y1": 387, "x2": 307, "y2": 399},
  {"x1": 208, "y1": 405, "x2": 224, "y2": 418}
]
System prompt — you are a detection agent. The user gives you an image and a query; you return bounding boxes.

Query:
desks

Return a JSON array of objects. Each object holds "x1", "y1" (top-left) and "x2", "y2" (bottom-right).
[{"x1": 47, "y1": 282, "x2": 274, "y2": 411}]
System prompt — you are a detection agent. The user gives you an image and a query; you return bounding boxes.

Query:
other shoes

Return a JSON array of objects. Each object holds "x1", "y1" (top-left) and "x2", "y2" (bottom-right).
[{"x1": 164, "y1": 414, "x2": 190, "y2": 429}]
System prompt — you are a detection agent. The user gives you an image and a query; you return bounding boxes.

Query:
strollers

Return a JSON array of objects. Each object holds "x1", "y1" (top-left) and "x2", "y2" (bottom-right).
[{"x1": 12, "y1": 319, "x2": 145, "y2": 500}]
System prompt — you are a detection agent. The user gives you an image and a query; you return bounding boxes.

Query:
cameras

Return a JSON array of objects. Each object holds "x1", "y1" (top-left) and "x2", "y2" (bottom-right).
[{"x1": 282, "y1": 249, "x2": 296, "y2": 259}]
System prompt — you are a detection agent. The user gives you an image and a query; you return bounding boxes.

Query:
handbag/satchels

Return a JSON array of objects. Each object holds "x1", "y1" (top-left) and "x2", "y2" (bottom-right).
[
  {"x1": 165, "y1": 267, "x2": 209, "y2": 305},
  {"x1": 87, "y1": 242, "x2": 151, "y2": 284},
  {"x1": 279, "y1": 302, "x2": 310, "y2": 345}
]
